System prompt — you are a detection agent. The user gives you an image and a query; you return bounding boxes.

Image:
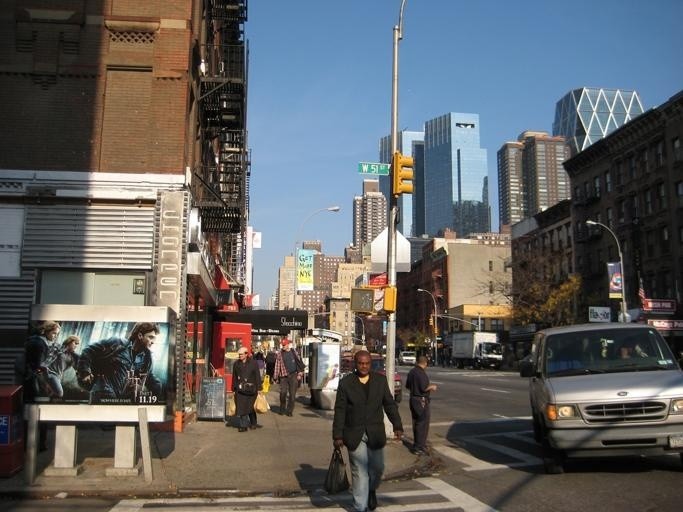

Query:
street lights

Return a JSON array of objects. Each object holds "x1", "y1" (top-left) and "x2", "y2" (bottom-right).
[
  {"x1": 292, "y1": 203, "x2": 342, "y2": 310},
  {"x1": 414, "y1": 288, "x2": 438, "y2": 366},
  {"x1": 350, "y1": 312, "x2": 368, "y2": 350},
  {"x1": 586, "y1": 218, "x2": 628, "y2": 321}
]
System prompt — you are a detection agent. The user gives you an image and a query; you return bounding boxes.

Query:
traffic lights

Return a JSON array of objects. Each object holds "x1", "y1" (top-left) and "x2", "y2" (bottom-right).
[{"x1": 392, "y1": 150, "x2": 415, "y2": 195}]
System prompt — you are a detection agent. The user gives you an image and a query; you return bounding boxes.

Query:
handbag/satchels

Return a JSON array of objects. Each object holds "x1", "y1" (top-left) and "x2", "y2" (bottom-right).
[{"x1": 239, "y1": 382, "x2": 257, "y2": 396}]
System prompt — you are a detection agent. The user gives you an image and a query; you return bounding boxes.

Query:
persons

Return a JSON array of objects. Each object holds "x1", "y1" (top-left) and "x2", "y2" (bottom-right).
[
  {"x1": 254, "y1": 348, "x2": 277, "y2": 385},
  {"x1": 14, "y1": 322, "x2": 61, "y2": 403},
  {"x1": 79, "y1": 323, "x2": 162, "y2": 404},
  {"x1": 333, "y1": 351, "x2": 403, "y2": 510},
  {"x1": 232, "y1": 347, "x2": 263, "y2": 432},
  {"x1": 406, "y1": 356, "x2": 437, "y2": 456},
  {"x1": 274, "y1": 338, "x2": 306, "y2": 418},
  {"x1": 46, "y1": 336, "x2": 82, "y2": 400}
]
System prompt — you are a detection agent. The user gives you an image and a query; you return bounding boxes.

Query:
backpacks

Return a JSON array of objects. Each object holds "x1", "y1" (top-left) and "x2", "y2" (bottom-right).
[{"x1": 324, "y1": 444, "x2": 352, "y2": 494}]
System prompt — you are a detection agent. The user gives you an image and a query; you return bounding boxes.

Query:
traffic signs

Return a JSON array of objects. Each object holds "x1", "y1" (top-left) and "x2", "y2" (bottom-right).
[{"x1": 356, "y1": 162, "x2": 391, "y2": 176}]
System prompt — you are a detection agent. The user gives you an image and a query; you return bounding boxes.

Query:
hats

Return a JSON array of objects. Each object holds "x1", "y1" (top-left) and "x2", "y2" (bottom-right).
[
  {"x1": 238, "y1": 346, "x2": 248, "y2": 355},
  {"x1": 281, "y1": 338, "x2": 288, "y2": 345}
]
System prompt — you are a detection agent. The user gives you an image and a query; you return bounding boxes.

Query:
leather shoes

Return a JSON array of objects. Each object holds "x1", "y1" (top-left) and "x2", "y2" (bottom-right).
[
  {"x1": 414, "y1": 447, "x2": 431, "y2": 455},
  {"x1": 368, "y1": 490, "x2": 378, "y2": 510},
  {"x1": 287, "y1": 409, "x2": 292, "y2": 417},
  {"x1": 239, "y1": 427, "x2": 248, "y2": 432},
  {"x1": 279, "y1": 408, "x2": 285, "y2": 415},
  {"x1": 413, "y1": 442, "x2": 428, "y2": 451},
  {"x1": 250, "y1": 424, "x2": 263, "y2": 429}
]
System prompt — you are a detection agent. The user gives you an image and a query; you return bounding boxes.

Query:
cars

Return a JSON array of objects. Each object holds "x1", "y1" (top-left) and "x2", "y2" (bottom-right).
[
  {"x1": 396, "y1": 349, "x2": 416, "y2": 365},
  {"x1": 517, "y1": 320, "x2": 682, "y2": 473}
]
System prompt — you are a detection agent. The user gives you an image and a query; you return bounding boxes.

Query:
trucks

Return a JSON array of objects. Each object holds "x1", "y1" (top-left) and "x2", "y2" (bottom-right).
[{"x1": 450, "y1": 329, "x2": 505, "y2": 371}]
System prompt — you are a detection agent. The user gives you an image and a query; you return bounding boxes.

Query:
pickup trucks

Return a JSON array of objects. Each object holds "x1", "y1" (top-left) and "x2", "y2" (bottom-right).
[{"x1": 336, "y1": 353, "x2": 403, "y2": 403}]
